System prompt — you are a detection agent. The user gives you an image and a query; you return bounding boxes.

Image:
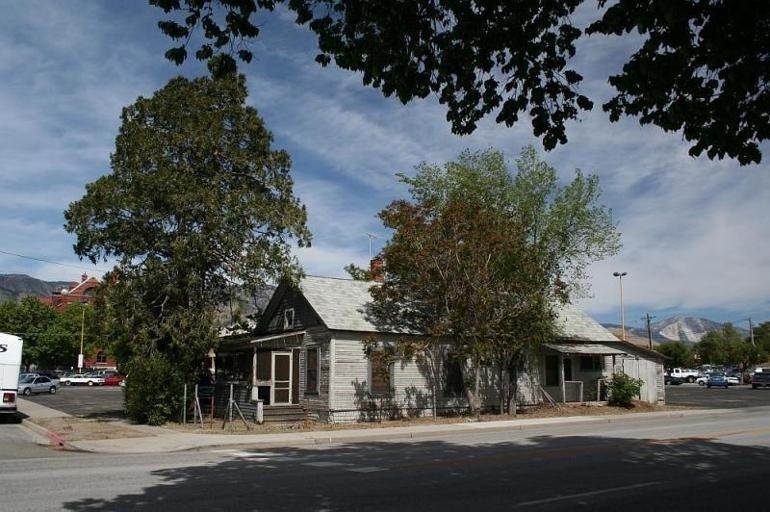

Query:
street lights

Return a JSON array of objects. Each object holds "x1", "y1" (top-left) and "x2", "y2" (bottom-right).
[
  {"x1": 78, "y1": 301, "x2": 89, "y2": 373},
  {"x1": 613, "y1": 271, "x2": 628, "y2": 341}
]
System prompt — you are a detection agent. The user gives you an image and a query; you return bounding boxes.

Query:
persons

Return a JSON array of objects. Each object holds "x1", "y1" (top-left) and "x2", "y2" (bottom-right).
[
  {"x1": 29, "y1": 362, "x2": 38, "y2": 373},
  {"x1": 70, "y1": 364, "x2": 74, "y2": 373}
]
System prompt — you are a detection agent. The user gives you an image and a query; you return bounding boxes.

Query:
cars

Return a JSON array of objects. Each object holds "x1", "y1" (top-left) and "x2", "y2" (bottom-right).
[
  {"x1": 18, "y1": 366, "x2": 126, "y2": 396},
  {"x1": 663, "y1": 364, "x2": 770, "y2": 389}
]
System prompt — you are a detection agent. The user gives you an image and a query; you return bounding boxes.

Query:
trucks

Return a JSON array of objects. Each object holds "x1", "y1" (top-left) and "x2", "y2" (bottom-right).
[{"x1": 0, "y1": 333, "x2": 23, "y2": 413}]
